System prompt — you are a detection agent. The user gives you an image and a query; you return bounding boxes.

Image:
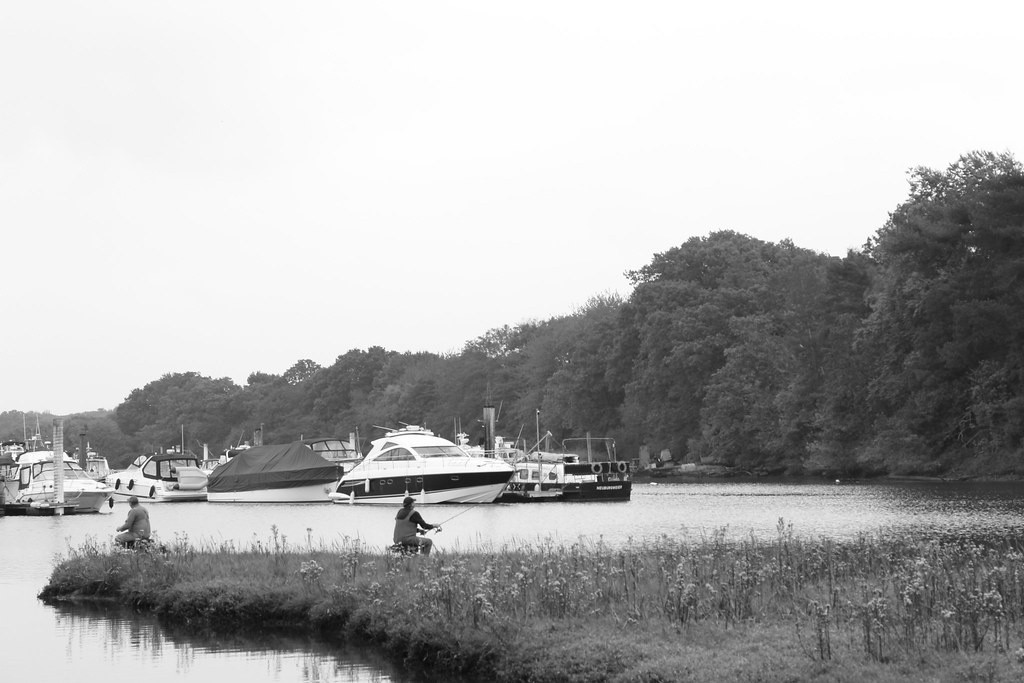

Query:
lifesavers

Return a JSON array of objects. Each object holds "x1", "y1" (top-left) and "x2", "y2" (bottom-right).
[
  {"x1": 617, "y1": 462, "x2": 628, "y2": 474},
  {"x1": 591, "y1": 463, "x2": 603, "y2": 474}
]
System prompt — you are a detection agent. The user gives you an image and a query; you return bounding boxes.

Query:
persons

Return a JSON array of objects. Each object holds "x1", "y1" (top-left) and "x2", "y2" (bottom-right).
[
  {"x1": 393, "y1": 497, "x2": 438, "y2": 557},
  {"x1": 115, "y1": 496, "x2": 151, "y2": 548}
]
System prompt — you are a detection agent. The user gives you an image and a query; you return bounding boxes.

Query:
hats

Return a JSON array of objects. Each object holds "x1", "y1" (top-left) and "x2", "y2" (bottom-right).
[
  {"x1": 403, "y1": 496, "x2": 416, "y2": 506},
  {"x1": 127, "y1": 496, "x2": 138, "y2": 503}
]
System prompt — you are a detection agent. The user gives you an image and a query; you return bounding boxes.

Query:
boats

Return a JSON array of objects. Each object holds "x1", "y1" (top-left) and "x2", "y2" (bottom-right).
[{"x1": 0, "y1": 406, "x2": 634, "y2": 515}]
